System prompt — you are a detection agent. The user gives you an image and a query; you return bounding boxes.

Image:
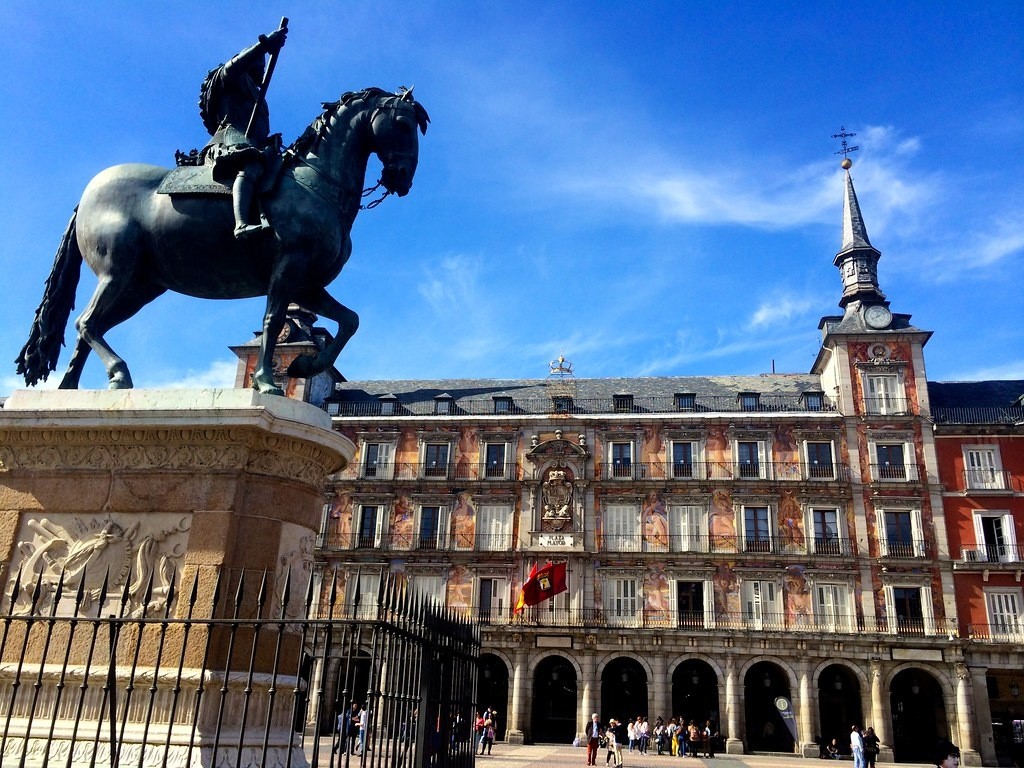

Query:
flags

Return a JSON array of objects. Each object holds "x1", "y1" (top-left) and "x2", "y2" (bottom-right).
[{"x1": 514, "y1": 561, "x2": 568, "y2": 615}]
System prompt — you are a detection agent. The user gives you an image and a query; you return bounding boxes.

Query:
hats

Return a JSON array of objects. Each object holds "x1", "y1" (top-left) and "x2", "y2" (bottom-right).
[{"x1": 609, "y1": 719, "x2": 616, "y2": 723}]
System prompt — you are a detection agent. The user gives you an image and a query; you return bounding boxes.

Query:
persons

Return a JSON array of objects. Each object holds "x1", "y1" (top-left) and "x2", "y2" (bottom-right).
[
  {"x1": 932, "y1": 741, "x2": 961, "y2": 768},
  {"x1": 405, "y1": 706, "x2": 498, "y2": 757},
  {"x1": 198, "y1": 27, "x2": 289, "y2": 240},
  {"x1": 585, "y1": 712, "x2": 602, "y2": 766},
  {"x1": 826, "y1": 725, "x2": 880, "y2": 768},
  {"x1": 605, "y1": 718, "x2": 627, "y2": 768},
  {"x1": 628, "y1": 716, "x2": 719, "y2": 758},
  {"x1": 333, "y1": 702, "x2": 371, "y2": 756}
]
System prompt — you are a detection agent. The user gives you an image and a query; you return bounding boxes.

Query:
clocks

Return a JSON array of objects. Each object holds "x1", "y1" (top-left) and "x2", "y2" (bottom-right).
[{"x1": 864, "y1": 306, "x2": 893, "y2": 330}]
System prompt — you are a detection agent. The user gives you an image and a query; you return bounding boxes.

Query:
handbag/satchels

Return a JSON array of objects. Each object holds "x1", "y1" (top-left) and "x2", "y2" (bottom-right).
[{"x1": 487, "y1": 727, "x2": 493, "y2": 738}]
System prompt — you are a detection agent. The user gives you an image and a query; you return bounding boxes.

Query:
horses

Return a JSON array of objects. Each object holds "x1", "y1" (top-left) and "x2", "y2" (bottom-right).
[{"x1": 15, "y1": 85, "x2": 431, "y2": 395}]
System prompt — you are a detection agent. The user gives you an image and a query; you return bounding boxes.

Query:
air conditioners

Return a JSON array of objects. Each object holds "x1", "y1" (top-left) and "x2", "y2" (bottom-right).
[{"x1": 962, "y1": 549, "x2": 983, "y2": 562}]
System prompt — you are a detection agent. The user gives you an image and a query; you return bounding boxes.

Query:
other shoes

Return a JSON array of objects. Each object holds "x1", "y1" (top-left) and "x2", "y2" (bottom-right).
[
  {"x1": 592, "y1": 764, "x2": 597, "y2": 766},
  {"x1": 615, "y1": 764, "x2": 622, "y2": 768},
  {"x1": 644, "y1": 752, "x2": 647, "y2": 755},
  {"x1": 629, "y1": 751, "x2": 634, "y2": 754},
  {"x1": 606, "y1": 763, "x2": 609, "y2": 766},
  {"x1": 480, "y1": 753, "x2": 484, "y2": 755},
  {"x1": 587, "y1": 763, "x2": 590, "y2": 766},
  {"x1": 488, "y1": 752, "x2": 490, "y2": 754},
  {"x1": 639, "y1": 752, "x2": 642, "y2": 755},
  {"x1": 670, "y1": 754, "x2": 715, "y2": 758}
]
